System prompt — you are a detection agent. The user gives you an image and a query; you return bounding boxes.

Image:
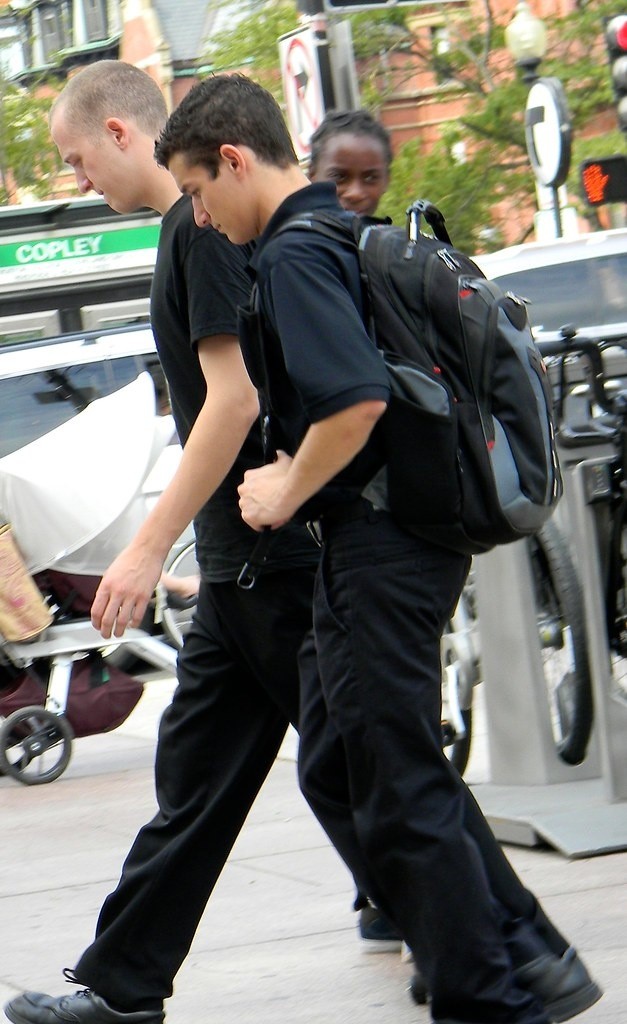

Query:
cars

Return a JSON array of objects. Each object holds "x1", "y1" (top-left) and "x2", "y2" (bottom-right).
[{"x1": 1, "y1": 319, "x2": 205, "y2": 648}]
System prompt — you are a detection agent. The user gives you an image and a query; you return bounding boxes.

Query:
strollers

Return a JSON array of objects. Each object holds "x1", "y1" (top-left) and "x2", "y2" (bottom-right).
[{"x1": 1, "y1": 372, "x2": 202, "y2": 786}]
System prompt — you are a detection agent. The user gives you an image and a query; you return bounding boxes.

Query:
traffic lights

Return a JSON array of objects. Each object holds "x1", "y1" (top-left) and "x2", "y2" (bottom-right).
[
  {"x1": 581, "y1": 155, "x2": 627, "y2": 204},
  {"x1": 602, "y1": 13, "x2": 627, "y2": 134}
]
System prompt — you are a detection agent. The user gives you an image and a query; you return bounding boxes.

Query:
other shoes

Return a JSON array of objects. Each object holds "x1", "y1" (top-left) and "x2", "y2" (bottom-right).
[{"x1": 355, "y1": 905, "x2": 413, "y2": 966}]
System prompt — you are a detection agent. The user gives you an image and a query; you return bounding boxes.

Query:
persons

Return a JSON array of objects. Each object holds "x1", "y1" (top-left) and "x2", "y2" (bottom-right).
[
  {"x1": 156, "y1": 74, "x2": 605, "y2": 1024},
  {"x1": 2, "y1": 61, "x2": 601, "y2": 1024}
]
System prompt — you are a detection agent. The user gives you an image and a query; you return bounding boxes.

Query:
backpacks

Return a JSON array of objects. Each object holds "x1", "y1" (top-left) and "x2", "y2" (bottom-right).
[{"x1": 236, "y1": 197, "x2": 566, "y2": 593}]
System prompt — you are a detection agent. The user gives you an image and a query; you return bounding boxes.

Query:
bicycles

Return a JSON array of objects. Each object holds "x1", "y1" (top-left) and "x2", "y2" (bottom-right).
[{"x1": 431, "y1": 321, "x2": 626, "y2": 777}]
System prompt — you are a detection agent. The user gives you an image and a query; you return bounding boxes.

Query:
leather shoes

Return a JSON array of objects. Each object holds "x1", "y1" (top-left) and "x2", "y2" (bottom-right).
[
  {"x1": 4, "y1": 965, "x2": 166, "y2": 1024},
  {"x1": 512, "y1": 944, "x2": 605, "y2": 1024}
]
